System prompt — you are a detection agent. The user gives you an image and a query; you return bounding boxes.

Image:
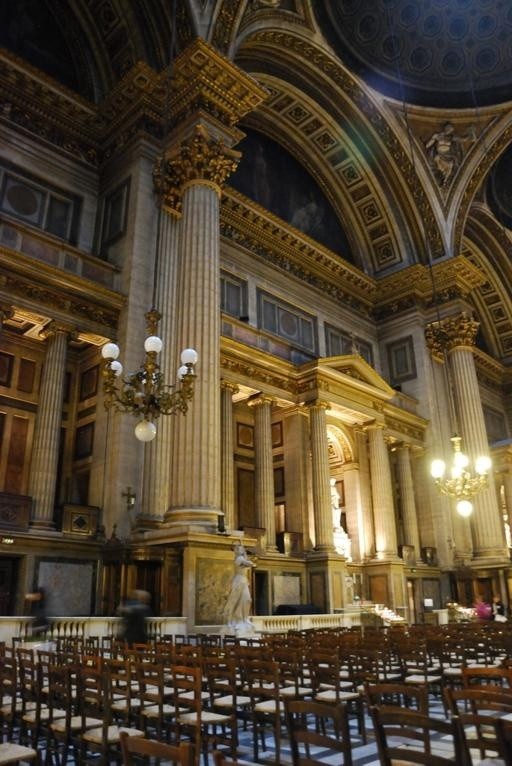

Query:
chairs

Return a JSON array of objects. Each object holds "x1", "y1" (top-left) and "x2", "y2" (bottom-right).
[{"x1": 1, "y1": 618, "x2": 512, "y2": 766}]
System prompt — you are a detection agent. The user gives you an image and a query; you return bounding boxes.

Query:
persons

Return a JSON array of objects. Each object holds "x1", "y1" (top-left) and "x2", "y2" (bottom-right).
[
  {"x1": 492, "y1": 595, "x2": 507, "y2": 622},
  {"x1": 331, "y1": 477, "x2": 340, "y2": 533},
  {"x1": 115, "y1": 590, "x2": 166, "y2": 651},
  {"x1": 223, "y1": 545, "x2": 255, "y2": 629}
]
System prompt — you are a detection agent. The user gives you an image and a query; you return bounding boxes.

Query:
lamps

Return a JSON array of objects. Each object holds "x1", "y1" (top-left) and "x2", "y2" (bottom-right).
[
  {"x1": 428, "y1": 329, "x2": 492, "y2": 519},
  {"x1": 100, "y1": 200, "x2": 199, "y2": 442}
]
